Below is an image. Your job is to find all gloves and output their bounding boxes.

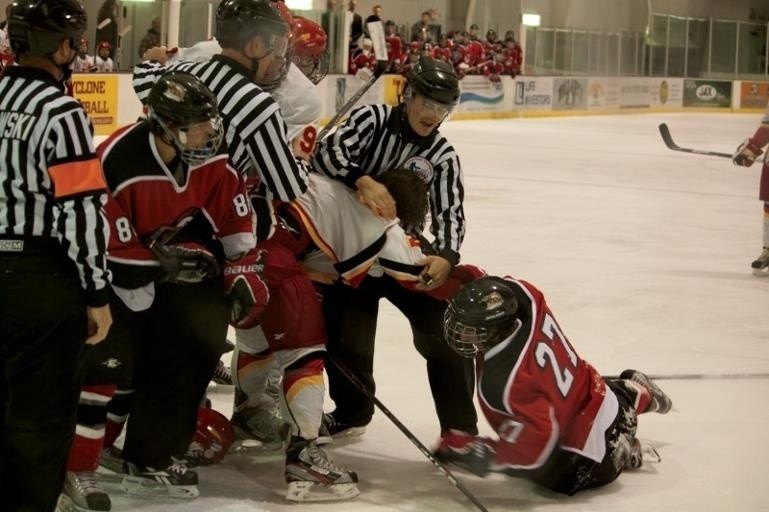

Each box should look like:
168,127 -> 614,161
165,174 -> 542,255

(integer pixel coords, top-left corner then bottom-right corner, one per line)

433,433 -> 494,478
174,242 -> 218,277
220,249 -> 270,329
732,137 -> 761,167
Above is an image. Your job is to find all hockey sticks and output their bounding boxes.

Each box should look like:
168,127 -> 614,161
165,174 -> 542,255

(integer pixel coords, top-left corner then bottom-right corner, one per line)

327,354 -> 489,512
314,15 -> 389,140
659,124 -> 764,164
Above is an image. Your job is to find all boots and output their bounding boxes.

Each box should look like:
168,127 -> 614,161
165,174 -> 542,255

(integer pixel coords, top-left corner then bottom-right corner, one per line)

123,458 -> 199,487
229,407 -> 290,444
751,247 -> 769,268
97,444 -> 127,472
324,408 -> 369,435
620,370 -> 671,414
62,469 -> 112,511
284,434 -> 359,484
212,361 -> 233,386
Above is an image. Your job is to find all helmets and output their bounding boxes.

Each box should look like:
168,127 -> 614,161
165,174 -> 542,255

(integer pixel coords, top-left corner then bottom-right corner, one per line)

98,42 -> 113,54
404,59 -> 460,104
80,39 -> 89,48
214,0 -> 294,46
290,15 -> 328,61
441,278 -> 519,358
6,0 -> 87,56
409,24 -> 515,54
148,71 -> 224,164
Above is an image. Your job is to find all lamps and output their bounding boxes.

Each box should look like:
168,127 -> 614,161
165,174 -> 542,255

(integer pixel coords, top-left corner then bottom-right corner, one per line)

521,11 -> 543,30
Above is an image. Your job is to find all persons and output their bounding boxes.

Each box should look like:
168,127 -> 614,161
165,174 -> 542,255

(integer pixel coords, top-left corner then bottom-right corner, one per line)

428,264 -> 672,493
734,103 -> 768,272
